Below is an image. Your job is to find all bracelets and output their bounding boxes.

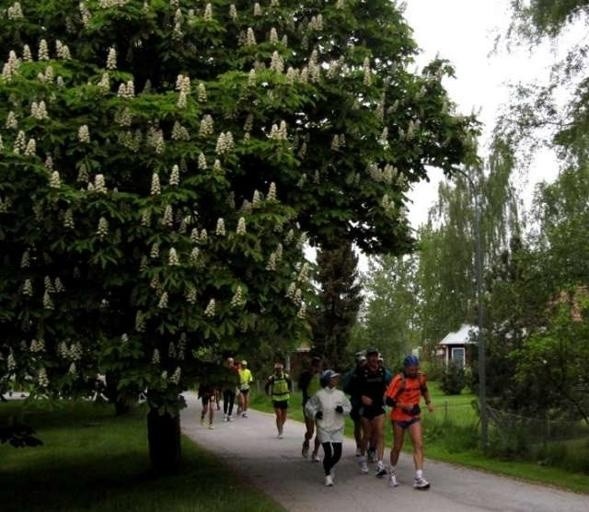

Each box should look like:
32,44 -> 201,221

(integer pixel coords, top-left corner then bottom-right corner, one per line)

425,400 -> 431,404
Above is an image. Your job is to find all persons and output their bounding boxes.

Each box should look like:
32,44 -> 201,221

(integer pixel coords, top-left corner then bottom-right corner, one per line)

196,356 -> 254,430
297,355 -> 323,464
383,354 -> 435,490
303,366 -> 353,487
342,346 -> 393,479
264,362 -> 296,440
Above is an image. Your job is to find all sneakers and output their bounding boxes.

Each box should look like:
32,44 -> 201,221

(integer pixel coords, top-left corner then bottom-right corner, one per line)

200,411 -> 249,430
302,441 -> 309,457
324,464 -> 336,486
312,450 -> 320,462
412,476 -> 430,490
277,432 -> 283,438
387,465 -> 400,487
356,446 -> 389,479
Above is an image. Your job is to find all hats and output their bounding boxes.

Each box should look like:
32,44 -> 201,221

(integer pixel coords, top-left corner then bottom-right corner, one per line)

321,369 -> 339,380
366,347 -> 379,356
354,352 -> 367,361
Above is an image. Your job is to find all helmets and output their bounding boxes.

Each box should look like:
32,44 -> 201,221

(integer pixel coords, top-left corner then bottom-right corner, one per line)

403,354 -> 419,366
